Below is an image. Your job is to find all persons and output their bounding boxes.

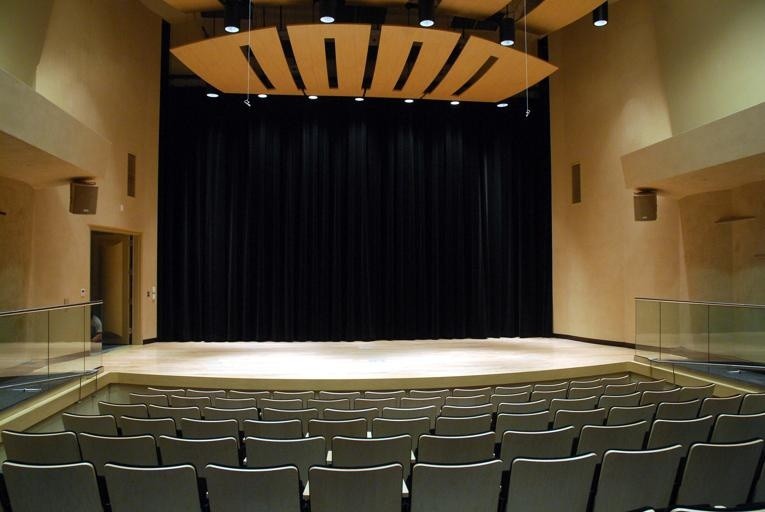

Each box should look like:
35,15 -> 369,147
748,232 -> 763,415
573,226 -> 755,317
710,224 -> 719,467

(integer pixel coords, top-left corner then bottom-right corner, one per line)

90,312 -> 102,342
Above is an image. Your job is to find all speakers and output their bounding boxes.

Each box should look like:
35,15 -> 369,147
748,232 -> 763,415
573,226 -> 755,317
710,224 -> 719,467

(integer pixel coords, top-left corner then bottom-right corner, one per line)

70,183 -> 99,215
633,192 -> 657,221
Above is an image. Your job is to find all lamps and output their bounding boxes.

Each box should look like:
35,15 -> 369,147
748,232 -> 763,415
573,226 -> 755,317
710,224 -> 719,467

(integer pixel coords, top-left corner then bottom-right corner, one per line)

319,0 -> 337,24
419,3 -> 437,31
592,2 -> 611,29
498,4 -> 516,48
224,2 -> 242,34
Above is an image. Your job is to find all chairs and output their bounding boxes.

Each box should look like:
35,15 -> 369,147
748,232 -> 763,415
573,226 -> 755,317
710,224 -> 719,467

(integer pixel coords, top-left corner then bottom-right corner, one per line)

272,391 -> 316,409
434,413 -> 493,437
370,405 -> 443,429
169,395 -> 212,417
606,382 -> 639,396
496,402 -> 549,416
308,398 -> 351,419
303,466 -> 410,512
204,406 -> 258,431
260,409 -> 319,439
569,379 -> 603,389
575,419 -> 651,457
712,412 -> 765,444
401,396 -> 445,419
159,435 -> 241,479
533,384 -> 570,393
506,452 -> 598,512
551,409 -> 606,430
494,411 -> 549,443
601,375 -> 631,386
740,394 -> 765,414
363,391 -> 406,409
530,389 -> 567,403
675,437 -> 765,512
638,380 -> 670,397
443,405 -> 491,416
332,435 -> 413,483
118,415 -> 178,447
647,415 -> 715,451
324,408 -> 379,434
128,392 -> 170,407
230,391 -> 270,409
98,399 -> 148,429
317,391 -> 362,410
640,388 -> 685,407
309,418 -> 369,450
215,397 -> 256,411
494,386 -> 532,397
678,385 -> 715,405
373,415 -> 433,450
242,419 -> 303,442
186,389 -> 226,407
262,398 -> 302,412
499,427 -> 577,472
353,399 -> 400,419
205,465 -> 300,512
146,404 -> 201,430
568,385 -> 605,406
453,389 -> 492,404
445,394 -> 487,408
607,404 -> 658,428
78,432 -> 159,476
600,392 -> 648,416
411,458 -> 506,512
549,396 -> 600,422
61,413 -> 120,437
0,461 -> 105,512
490,391 -> 529,412
656,399 -> 703,423
595,443 -> 683,512
0,428 -> 80,467
247,436 -> 329,488
148,387 -> 186,407
179,415 -> 240,451
699,394 -> 744,417
418,431 -> 496,467
408,389 -> 452,407
104,464 -> 202,512
